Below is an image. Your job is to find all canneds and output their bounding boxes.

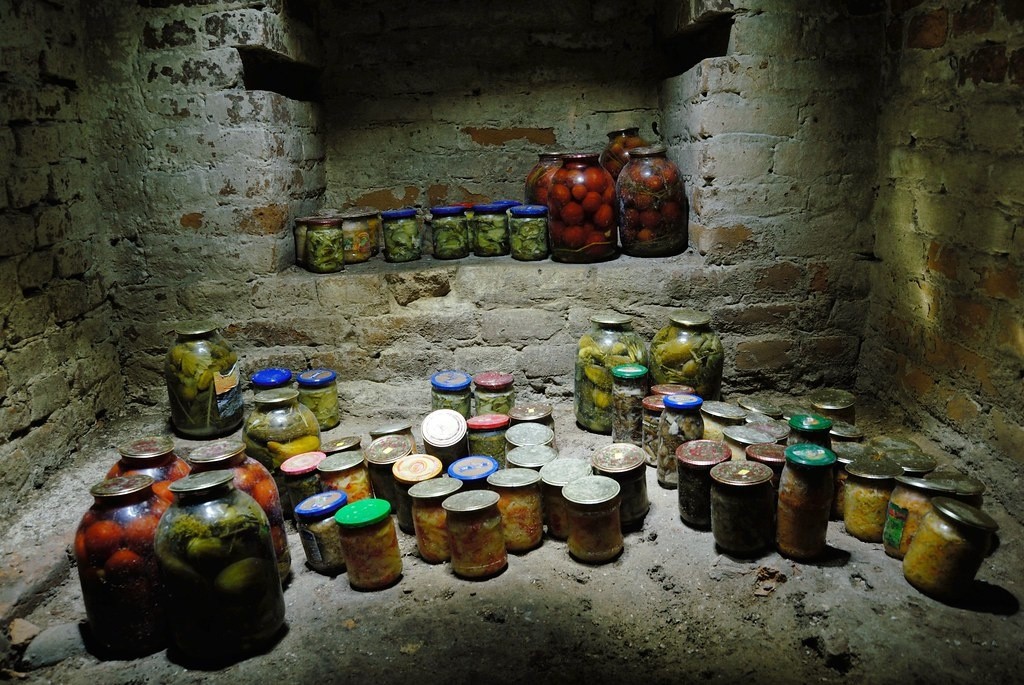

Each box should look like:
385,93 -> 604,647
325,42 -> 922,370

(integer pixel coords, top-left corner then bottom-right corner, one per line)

77,320 -> 995,662
295,127 -> 688,272
